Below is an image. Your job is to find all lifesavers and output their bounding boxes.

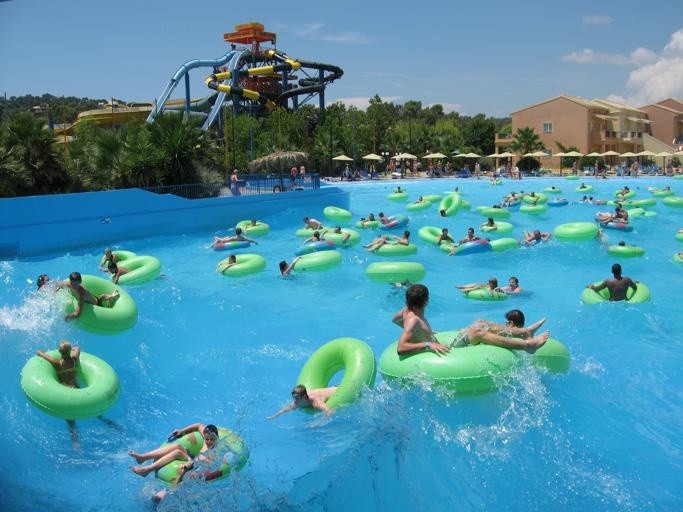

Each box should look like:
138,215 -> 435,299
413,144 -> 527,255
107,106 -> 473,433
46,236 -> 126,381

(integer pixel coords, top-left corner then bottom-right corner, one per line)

53,250 -> 161,335
217,253 -> 264,278
212,219 -> 269,251
292,226 -> 361,273
673,175 -> 683,179
406,184 -> 683,266
366,261 -> 425,285
567,176 -> 579,180
356,212 -> 417,256
388,188 -> 408,202
581,280 -> 651,307
460,285 -> 508,302
153,427 -> 250,486
20,348 -> 120,419
488,179 -> 502,185
295,331 -> 570,416
324,206 -> 352,223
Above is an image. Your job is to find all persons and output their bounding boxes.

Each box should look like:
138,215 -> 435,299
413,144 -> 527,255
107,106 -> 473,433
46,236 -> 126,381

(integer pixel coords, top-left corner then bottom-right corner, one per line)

493,276 -> 523,294
290,164 -> 298,186
368,212 -> 375,221
98,248 -> 121,272
455,277 -> 498,292
391,283 -> 550,364
250,218 -> 258,226
303,229 -> 322,244
585,263 -> 637,300
378,212 -> 389,224
209,225 -> 258,249
579,182 -> 633,246
478,216 -> 497,233
127,422 -> 219,479
571,159 -> 640,179
214,254 -> 236,272
475,309 -> 525,329
437,228 -> 455,244
522,227 -> 552,244
36,274 -> 51,289
54,271 -> 120,320
229,169 -> 241,196
475,161 -> 480,178
149,465 -> 185,503
394,183 -> 534,217
303,217 -> 322,230
34,341 -> 125,451
488,160 -> 522,186
108,262 -> 129,284
362,229 -> 411,251
462,227 -> 477,245
412,160 -> 453,180
266,384 -> 338,430
321,226 -> 351,244
279,255 -> 302,276
359,217 -> 368,227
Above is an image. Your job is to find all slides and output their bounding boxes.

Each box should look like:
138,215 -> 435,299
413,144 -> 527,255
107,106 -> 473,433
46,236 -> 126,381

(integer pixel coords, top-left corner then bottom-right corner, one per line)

205,50 -> 299,111
145,48 -> 250,141
278,57 -> 343,110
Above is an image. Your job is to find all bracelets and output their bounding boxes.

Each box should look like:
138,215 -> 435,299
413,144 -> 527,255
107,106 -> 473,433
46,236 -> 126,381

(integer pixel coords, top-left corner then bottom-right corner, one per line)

588,284 -> 593,289
424,341 -> 430,350
